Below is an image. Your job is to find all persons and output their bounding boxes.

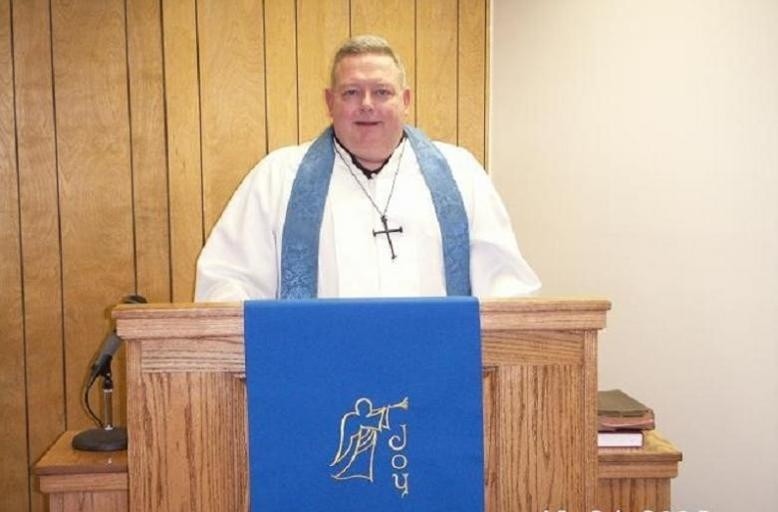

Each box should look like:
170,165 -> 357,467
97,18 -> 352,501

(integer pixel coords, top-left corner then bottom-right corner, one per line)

194,35 -> 542,302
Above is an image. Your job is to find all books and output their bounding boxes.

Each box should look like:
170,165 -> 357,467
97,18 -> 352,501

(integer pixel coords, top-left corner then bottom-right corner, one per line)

597,388 -> 655,448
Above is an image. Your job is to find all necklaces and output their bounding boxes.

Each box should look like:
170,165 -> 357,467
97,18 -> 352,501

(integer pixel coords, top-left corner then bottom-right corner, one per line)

332,134 -> 407,260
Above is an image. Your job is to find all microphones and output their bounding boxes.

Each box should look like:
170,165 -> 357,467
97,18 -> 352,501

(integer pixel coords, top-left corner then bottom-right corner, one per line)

86,294 -> 147,387
